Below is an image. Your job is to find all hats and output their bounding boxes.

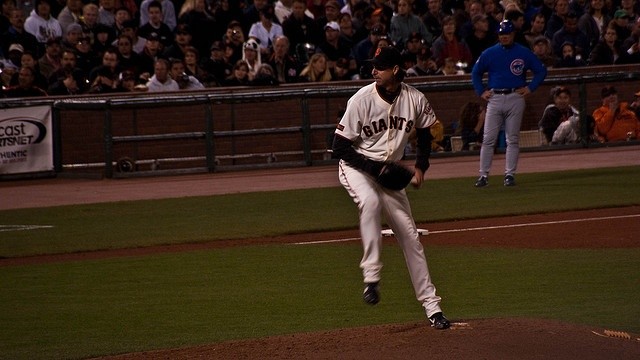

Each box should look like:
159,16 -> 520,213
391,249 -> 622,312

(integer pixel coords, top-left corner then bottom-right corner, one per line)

497,20 -> 514,32
600,85 -> 618,97
324,21 -> 340,30
408,30 -> 423,42
371,22 -> 386,36
243,37 -> 261,51
211,41 -> 226,53
364,47 -> 404,67
66,23 -> 83,35
470,14 -> 488,26
614,9 -> 629,18
325,1 -> 340,10
174,24 -> 191,34
8,43 -> 24,54
417,47 -> 434,61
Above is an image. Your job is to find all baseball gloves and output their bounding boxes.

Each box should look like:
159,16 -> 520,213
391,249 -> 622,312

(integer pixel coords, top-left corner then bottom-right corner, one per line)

358,155 -> 415,191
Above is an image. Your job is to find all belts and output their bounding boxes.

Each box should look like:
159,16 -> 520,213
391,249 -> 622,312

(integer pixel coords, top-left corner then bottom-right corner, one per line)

493,88 -> 516,95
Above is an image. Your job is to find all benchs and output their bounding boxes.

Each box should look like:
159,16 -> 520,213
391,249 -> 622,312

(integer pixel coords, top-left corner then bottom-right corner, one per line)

450,128 -> 545,153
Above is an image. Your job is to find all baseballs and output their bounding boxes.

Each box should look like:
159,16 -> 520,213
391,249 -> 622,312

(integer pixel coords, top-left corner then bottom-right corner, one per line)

411,176 -> 423,188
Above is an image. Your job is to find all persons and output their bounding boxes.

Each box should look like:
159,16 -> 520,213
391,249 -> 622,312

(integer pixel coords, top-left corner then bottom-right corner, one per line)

524,14 -> 551,46
313,0 -> 340,40
590,23 -> 629,65
551,11 -> 586,56
558,42 -> 587,67
406,47 -> 447,77
462,14 -> 493,73
100,46 -> 119,74
146,59 -> 179,91
206,41 -> 234,81
578,0 -> 609,42
503,4 -> 522,20
316,21 -> 342,60
608,9 -> 630,39
341,0 -> 372,14
24,1 -> 62,42
135,32 -> 169,77
339,13 -> 360,44
470,2 -> 484,21
592,87 -> 639,143
0,43 -> 26,71
267,36 -> 298,83
472,19 -> 549,189
298,53 -> 334,81
484,0 -> 504,21
112,22 -> 147,53
624,16 -> 640,49
618,0 -> 640,19
546,0 -> 570,37
400,32 -> 429,69
237,38 -> 263,81
222,20 -> 245,60
109,8 -> 138,42
629,88 -> 640,120
461,95 -> 488,144
408,115 -> 444,152
614,39 -> 639,64
162,24 -> 200,58
0,10 -> 42,64
432,15 -> 473,75
20,52 -> 37,75
59,23 -> 93,65
182,46 -> 208,88
504,8 -> 529,49
248,7 -> 284,54
331,57 -> 360,81
541,87 -> 582,144
48,49 -> 90,95
89,24 -> 118,67
531,36 -> 556,68
86,75 -> 113,93
76,4 -> 101,33
281,0 -> 317,54
179,0 -> 216,44
9,51 -> 49,93
350,24 -> 387,58
390,0 -> 434,46
242,0 -> 281,33
421,0 -> 447,36
48,69 -> 80,95
96,0 -> 118,25
251,63 -> 278,86
57,0 -> 83,33
138,1 -> 173,44
224,60 -> 251,86
140,0 -> 176,31
4,66 -> 49,97
0,0 -> 18,30
376,36 -> 389,52
117,35 -> 142,69
541,88 -> 580,126
274,0 -> 314,27
38,37 -> 65,77
170,59 -> 206,90
332,45 -> 451,330
119,69 -> 135,80
115,71 -> 148,92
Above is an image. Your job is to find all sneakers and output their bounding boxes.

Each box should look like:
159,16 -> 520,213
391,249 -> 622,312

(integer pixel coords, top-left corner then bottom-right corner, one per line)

475,175 -> 488,187
503,174 -> 514,186
429,313 -> 450,329
363,283 -> 381,305
